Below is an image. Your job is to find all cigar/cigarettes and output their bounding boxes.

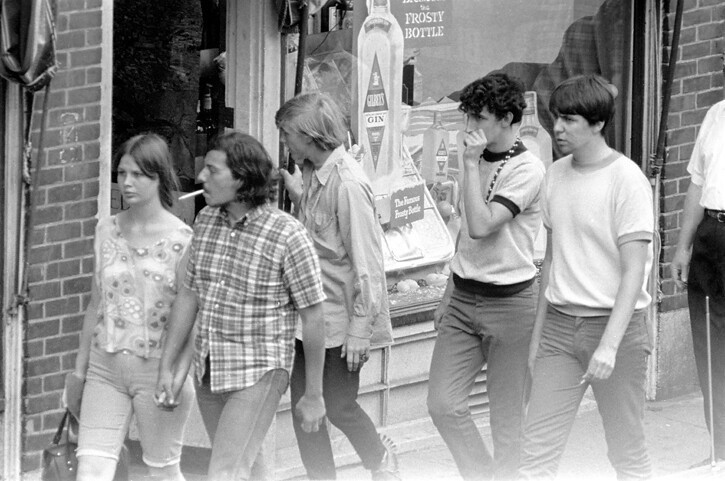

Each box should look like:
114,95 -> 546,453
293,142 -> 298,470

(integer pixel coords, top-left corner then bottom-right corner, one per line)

178,189 -> 204,200
581,379 -> 585,384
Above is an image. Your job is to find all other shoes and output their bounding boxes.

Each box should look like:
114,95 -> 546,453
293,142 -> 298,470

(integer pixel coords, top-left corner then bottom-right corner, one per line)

369,445 -> 396,480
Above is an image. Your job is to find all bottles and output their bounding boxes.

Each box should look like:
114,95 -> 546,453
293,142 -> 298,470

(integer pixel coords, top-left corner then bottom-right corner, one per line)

195,83 -> 219,156
421,110 -> 448,183
357,0 -> 404,194
517,90 -> 553,172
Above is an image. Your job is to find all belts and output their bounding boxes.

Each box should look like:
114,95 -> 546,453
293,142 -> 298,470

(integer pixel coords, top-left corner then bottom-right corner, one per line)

707,210 -> 725,223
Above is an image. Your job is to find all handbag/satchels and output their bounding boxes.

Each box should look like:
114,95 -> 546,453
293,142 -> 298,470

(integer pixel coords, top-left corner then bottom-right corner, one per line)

42,410 -> 131,481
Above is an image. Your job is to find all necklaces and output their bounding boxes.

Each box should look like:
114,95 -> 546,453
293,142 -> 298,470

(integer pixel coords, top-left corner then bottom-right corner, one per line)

478,136 -> 521,205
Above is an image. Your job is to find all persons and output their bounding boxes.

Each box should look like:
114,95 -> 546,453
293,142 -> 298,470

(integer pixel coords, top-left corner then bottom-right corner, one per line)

670,47 -> 725,469
61,132 -> 327,481
514,74 -> 654,481
274,90 -> 402,481
426,73 -> 547,481
